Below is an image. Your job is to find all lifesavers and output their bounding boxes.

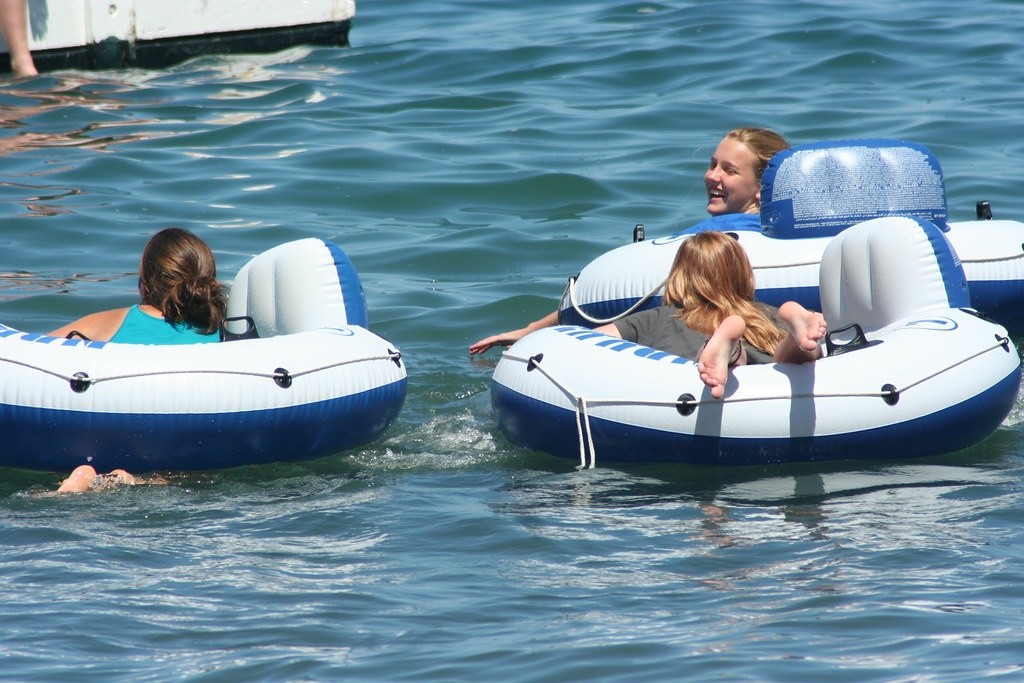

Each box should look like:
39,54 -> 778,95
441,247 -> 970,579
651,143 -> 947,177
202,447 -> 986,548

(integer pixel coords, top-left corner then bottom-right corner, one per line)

558,139 -> 1024,323
490,216 -> 1022,464
0,234 -> 409,475
4,3 -> 359,62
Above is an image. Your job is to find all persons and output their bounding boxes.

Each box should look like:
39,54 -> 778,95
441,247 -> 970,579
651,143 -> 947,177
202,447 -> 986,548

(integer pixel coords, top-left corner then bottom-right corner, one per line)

592,232 -> 827,399
0,0 -> 37,75
470,129 -> 789,354
47,227 -> 228,492
